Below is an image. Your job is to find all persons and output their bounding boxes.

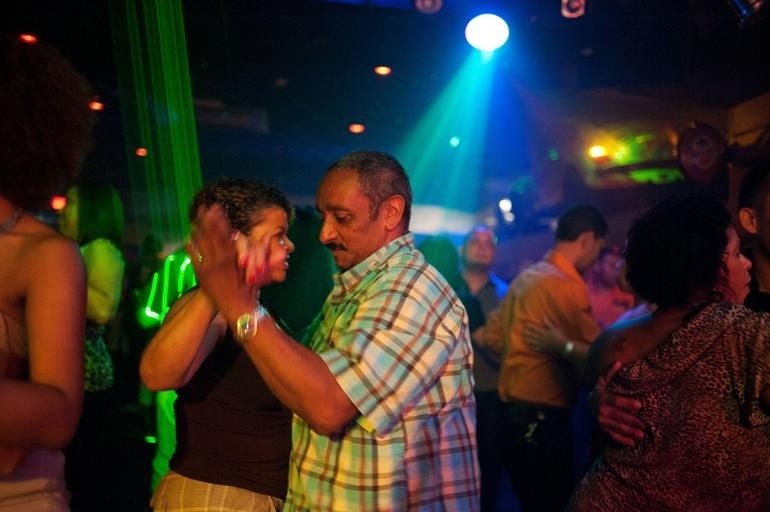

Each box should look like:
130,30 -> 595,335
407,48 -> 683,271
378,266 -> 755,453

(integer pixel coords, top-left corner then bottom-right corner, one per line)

1,38 -> 770,511
